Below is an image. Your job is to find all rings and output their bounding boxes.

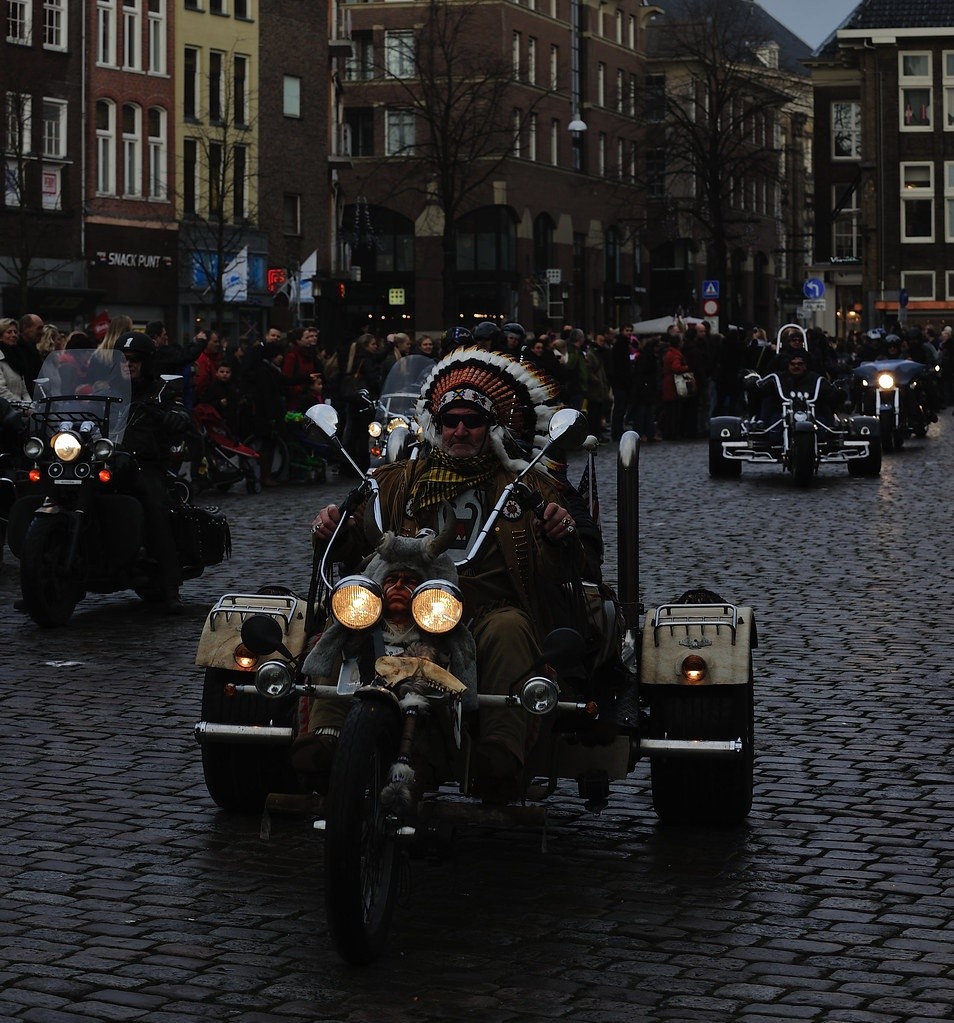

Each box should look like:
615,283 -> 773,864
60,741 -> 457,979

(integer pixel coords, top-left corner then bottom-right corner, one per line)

316,521 -> 323,529
311,527 -> 317,534
561,517 -> 569,527
567,525 -> 575,533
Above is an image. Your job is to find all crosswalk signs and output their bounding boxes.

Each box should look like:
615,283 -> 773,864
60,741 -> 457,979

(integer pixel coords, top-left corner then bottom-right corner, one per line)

699,279 -> 722,300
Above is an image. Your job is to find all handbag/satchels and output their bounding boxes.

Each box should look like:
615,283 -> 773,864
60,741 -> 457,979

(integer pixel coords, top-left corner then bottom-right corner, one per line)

674,371 -> 696,397
343,359 -> 380,397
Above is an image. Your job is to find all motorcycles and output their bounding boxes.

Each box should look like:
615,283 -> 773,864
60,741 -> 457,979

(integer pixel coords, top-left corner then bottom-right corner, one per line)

1,348 -> 235,631
846,359 -> 941,446
708,370 -> 883,488
354,354 -> 439,478
187,400 -> 761,966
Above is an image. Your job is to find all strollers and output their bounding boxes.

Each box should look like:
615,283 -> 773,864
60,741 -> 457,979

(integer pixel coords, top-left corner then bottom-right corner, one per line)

190,400 -> 265,498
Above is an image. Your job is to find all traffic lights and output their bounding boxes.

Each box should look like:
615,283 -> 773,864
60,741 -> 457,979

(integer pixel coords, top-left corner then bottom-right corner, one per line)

331,278 -> 350,303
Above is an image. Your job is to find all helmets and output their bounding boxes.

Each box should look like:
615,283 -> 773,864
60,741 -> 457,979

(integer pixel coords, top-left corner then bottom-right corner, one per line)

113,331 -> 158,362
475,321 -> 500,338
866,329 -> 881,339
877,328 -> 887,334
885,334 -> 902,349
452,327 -> 476,345
780,327 -> 804,344
502,322 -> 526,338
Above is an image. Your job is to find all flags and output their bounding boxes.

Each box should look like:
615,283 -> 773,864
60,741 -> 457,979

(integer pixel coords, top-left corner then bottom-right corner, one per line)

577,452 -> 602,530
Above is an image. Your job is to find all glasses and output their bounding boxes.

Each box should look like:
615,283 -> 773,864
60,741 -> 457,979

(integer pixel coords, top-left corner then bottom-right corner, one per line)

625,328 -> 632,332
791,362 -> 803,366
440,412 -> 489,432
120,354 -> 140,364
792,337 -> 803,342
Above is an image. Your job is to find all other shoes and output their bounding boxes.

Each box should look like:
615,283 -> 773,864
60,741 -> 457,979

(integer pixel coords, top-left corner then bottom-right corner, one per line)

587,431 -> 610,446
647,436 -> 663,444
639,437 -> 648,445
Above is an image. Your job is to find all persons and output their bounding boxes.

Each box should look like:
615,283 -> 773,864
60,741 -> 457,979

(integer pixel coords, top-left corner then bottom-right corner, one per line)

305,493 -> 479,709
0,309 -> 953,576
291,346 -> 604,797
